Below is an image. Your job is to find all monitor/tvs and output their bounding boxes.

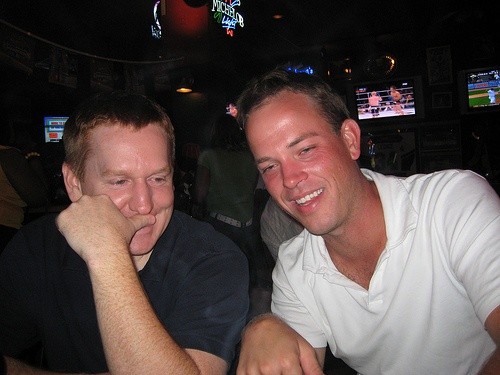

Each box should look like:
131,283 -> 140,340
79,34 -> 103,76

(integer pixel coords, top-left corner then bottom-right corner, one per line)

465,67 -> 500,108
353,78 -> 416,120
44,117 -> 70,143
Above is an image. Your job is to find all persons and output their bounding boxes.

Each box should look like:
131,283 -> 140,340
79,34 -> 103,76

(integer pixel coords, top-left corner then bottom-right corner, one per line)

488,88 -> 496,104
0,91 -> 250,375
390,86 -> 404,114
235,67 -> 500,375
493,73 -> 500,86
369,92 -> 382,117
193,114 -> 258,271
